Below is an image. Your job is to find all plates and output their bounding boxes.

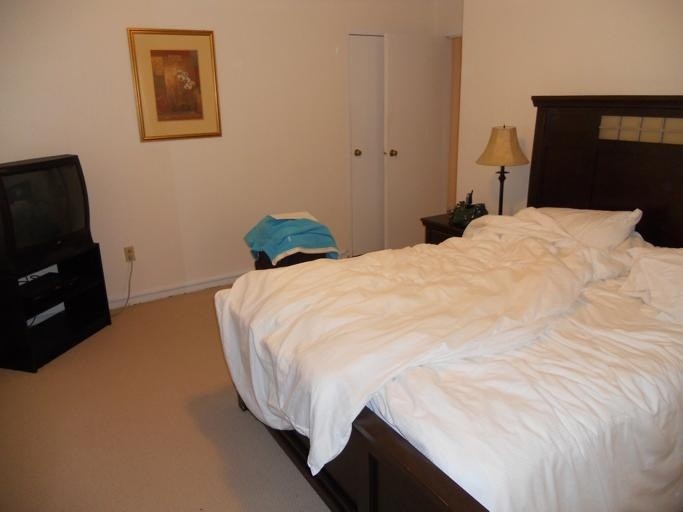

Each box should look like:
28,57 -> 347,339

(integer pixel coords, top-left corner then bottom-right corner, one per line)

0,242 -> 113,373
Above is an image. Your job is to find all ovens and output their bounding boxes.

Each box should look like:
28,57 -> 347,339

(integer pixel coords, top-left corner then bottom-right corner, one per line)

212,91 -> 681,511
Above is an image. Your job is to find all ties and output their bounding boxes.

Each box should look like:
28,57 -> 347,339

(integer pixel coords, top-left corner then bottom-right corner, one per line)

0,153 -> 94,271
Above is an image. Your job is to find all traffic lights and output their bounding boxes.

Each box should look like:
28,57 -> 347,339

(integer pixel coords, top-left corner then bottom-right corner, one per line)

619,246 -> 681,319
535,207 -> 643,250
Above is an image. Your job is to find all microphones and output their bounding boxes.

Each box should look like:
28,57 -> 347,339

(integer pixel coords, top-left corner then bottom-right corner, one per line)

252,245 -> 325,270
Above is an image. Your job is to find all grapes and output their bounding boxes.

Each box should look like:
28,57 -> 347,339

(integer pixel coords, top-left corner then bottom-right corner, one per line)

420,211 -> 485,246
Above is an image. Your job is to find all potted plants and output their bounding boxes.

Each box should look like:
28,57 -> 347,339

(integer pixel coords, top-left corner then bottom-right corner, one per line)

125,27 -> 223,144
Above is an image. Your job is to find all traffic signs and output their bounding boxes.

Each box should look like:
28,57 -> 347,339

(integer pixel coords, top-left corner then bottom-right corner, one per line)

122,247 -> 136,263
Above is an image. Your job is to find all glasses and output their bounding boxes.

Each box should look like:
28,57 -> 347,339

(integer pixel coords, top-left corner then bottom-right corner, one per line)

475,124 -> 531,215
